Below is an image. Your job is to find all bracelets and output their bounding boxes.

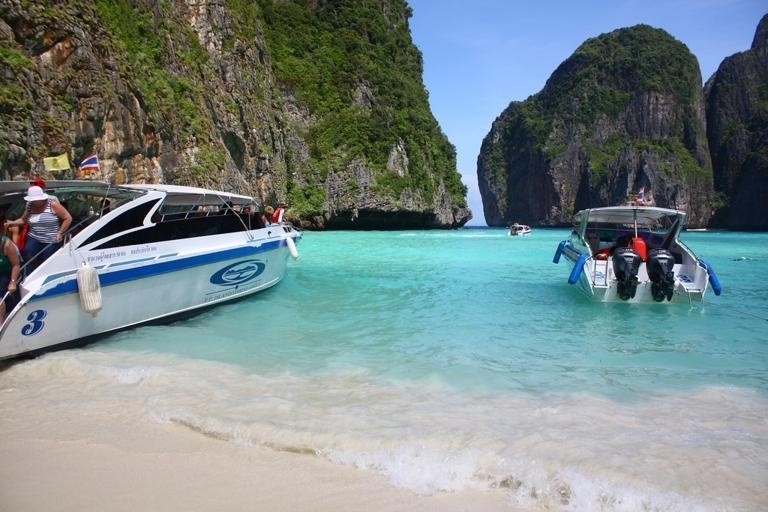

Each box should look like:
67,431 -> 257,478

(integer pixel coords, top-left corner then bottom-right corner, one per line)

10,279 -> 17,283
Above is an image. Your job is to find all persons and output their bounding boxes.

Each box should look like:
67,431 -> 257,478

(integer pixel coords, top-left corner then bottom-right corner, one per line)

270,204 -> 288,225
244,206 -> 253,216
6,178 -> 48,251
262,206 -> 274,226
2,186 -> 72,277
1,235 -> 26,322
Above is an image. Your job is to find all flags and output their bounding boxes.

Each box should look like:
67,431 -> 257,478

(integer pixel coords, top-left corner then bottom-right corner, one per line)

43,152 -> 71,173
78,151 -> 99,172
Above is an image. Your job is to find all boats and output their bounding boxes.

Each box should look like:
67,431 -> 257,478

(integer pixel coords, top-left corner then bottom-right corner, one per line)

0,170 -> 304,362
507,223 -> 531,238
556,203 -> 724,314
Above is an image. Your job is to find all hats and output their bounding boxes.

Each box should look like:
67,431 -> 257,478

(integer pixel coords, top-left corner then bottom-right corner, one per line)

264,206 -> 274,213
24,178 -> 49,201
277,203 -> 288,208
